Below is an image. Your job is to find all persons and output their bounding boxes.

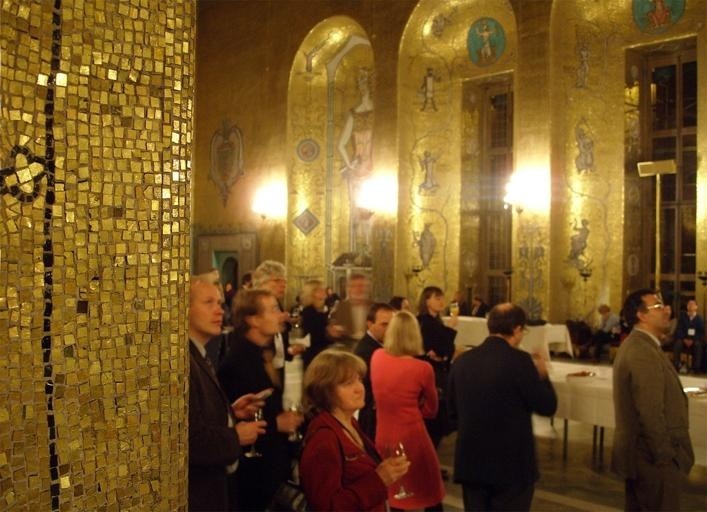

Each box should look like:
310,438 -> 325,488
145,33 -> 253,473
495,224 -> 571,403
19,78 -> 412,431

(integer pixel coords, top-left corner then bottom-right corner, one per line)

414,285 -> 458,448
298,348 -> 411,512
583,305 -> 630,363
224,282 -> 235,303
188,277 -> 267,512
327,275 -> 375,341
389,296 -> 412,312
369,312 -> 446,512
240,273 -> 252,290
301,280 -> 331,367
442,302 -> 559,512
252,260 -> 304,394
472,297 -> 490,318
216,289 -> 304,489
353,303 -> 395,438
325,286 -> 341,309
609,289 -> 695,512
661,299 -> 703,374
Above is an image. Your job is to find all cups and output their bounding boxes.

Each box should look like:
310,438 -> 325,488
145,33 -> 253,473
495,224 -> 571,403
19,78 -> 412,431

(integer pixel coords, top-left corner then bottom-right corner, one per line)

384,438 -> 415,499
449,302 -> 459,320
244,408 -> 263,458
287,400 -> 304,440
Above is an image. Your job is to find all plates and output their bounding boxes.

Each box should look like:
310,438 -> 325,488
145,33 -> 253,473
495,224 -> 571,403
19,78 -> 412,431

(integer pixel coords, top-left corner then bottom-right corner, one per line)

566,372 -> 596,382
683,387 -> 707,397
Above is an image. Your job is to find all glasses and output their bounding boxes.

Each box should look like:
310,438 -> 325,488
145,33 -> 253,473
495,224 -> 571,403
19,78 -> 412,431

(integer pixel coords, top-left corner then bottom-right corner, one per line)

270,278 -> 288,283
522,325 -> 531,334
646,302 -> 666,311
